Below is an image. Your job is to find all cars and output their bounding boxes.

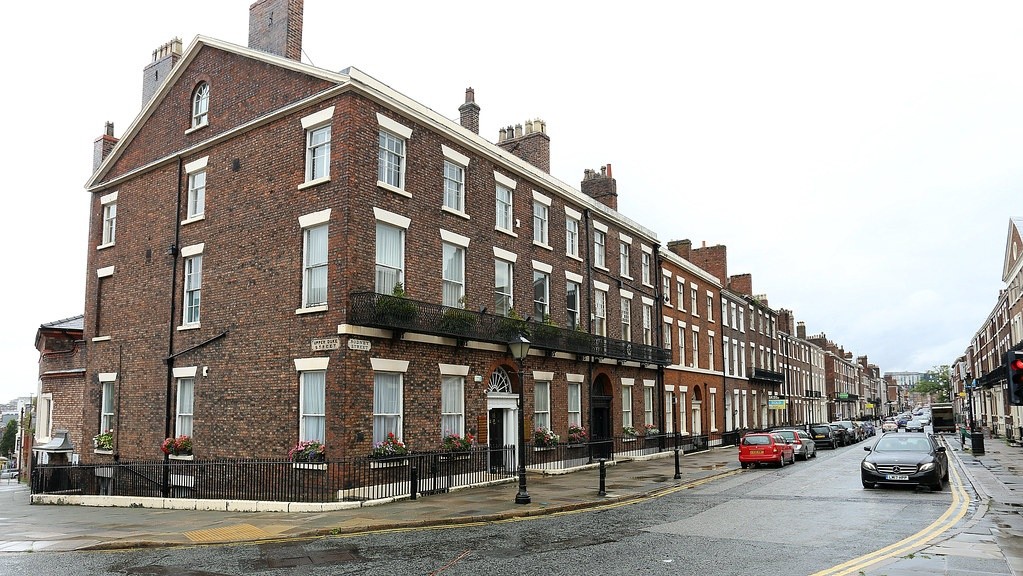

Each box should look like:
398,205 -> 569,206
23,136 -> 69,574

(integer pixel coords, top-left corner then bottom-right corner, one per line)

882,421 -> 899,432
912,404 -> 932,425
897,414 -> 912,428
905,421 -> 924,433
829,424 -> 851,446
836,421 -> 876,443
769,429 -> 817,461
11,461 -> 17,468
861,432 -> 949,491
809,426 -> 838,450
2,469 -> 19,479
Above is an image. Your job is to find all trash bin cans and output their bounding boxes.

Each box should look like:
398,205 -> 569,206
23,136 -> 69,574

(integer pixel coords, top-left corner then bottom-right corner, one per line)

972,431 -> 985,453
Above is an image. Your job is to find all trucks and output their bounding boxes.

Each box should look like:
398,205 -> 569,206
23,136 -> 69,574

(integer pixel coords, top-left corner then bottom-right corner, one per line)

930,403 -> 956,433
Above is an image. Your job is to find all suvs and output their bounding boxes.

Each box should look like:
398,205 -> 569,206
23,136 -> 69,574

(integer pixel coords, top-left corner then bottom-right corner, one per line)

739,431 -> 794,468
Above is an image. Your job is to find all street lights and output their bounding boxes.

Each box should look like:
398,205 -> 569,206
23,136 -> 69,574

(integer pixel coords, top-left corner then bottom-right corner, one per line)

507,331 -> 532,504
964,373 -> 974,433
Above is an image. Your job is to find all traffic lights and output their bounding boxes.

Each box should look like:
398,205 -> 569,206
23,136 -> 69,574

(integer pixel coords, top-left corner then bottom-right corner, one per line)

1006,350 -> 1023,406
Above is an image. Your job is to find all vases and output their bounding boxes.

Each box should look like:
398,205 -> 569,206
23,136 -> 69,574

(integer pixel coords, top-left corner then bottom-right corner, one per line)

375,452 -> 408,463
295,450 -> 325,462
171,448 -> 192,456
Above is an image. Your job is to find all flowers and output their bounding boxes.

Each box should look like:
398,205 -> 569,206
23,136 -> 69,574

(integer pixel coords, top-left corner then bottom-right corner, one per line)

644,424 -> 660,434
368,432 -> 410,459
569,423 -> 588,444
160,433 -> 192,455
535,425 -> 559,447
440,427 -> 476,451
93,429 -> 114,451
288,439 -> 325,462
